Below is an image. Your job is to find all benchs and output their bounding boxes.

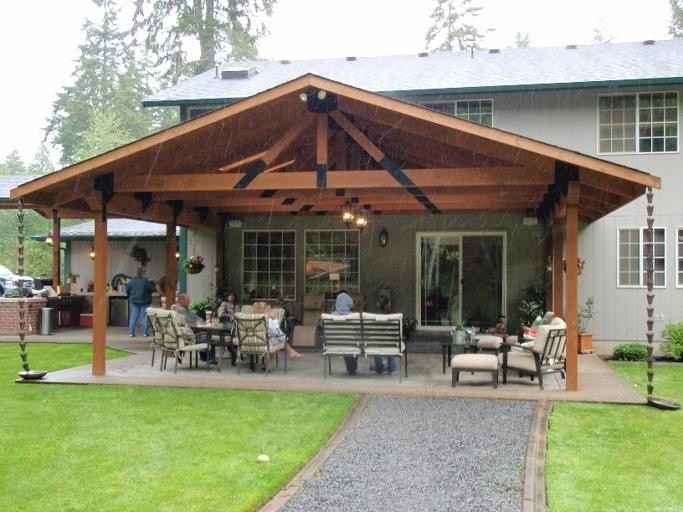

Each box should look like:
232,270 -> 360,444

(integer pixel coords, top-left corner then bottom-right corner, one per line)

320,313 -> 407,383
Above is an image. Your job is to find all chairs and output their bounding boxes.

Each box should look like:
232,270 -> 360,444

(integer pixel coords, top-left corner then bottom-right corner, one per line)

154,313 -> 212,374
496,329 -> 566,389
521,317 -> 566,350
145,310 -> 189,366
233,314 -> 288,378
518,312 -> 554,342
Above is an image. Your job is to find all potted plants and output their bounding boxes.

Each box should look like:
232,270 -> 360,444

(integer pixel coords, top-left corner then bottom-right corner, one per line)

563,257 -> 584,276
181,255 -> 205,274
578,296 -> 595,354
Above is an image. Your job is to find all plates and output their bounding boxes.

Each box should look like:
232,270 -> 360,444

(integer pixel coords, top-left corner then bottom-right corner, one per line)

648,397 -> 680,409
18,370 -> 48,379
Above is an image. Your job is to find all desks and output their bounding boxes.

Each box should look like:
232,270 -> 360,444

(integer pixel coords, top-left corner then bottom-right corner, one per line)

440,338 -> 508,373
186,321 -> 265,373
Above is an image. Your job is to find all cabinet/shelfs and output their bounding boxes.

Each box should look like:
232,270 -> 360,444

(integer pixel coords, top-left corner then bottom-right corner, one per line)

303,295 -> 364,326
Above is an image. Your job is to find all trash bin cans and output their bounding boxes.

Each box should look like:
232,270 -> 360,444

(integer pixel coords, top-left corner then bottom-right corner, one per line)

39,308 -> 54,336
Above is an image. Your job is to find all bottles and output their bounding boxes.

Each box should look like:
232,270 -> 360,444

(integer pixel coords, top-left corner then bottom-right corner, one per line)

107,282 -> 109,289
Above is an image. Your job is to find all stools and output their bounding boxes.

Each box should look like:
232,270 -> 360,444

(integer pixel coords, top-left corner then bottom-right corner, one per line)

451,354 -> 499,388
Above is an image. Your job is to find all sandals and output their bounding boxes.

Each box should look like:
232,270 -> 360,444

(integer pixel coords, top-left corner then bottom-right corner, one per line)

289,352 -> 303,359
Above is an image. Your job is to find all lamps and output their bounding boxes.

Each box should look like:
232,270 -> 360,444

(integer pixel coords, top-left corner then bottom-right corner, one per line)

90,237 -> 96,259
355,209 -> 369,233
380,226 -> 388,246
342,203 -> 355,227
45,232 -> 53,245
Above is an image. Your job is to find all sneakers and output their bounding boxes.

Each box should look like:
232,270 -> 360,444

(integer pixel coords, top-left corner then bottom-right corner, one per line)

209,361 -> 218,365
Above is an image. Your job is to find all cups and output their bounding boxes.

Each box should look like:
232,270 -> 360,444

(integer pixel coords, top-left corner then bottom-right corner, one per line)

206,310 -> 212,323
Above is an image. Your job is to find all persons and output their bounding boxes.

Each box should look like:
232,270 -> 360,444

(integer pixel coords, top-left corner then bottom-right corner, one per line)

219,290 -> 238,323
370,295 -> 398,374
171,294 -> 217,366
253,302 -> 304,372
126,267 -> 157,337
331,292 -> 361,375
